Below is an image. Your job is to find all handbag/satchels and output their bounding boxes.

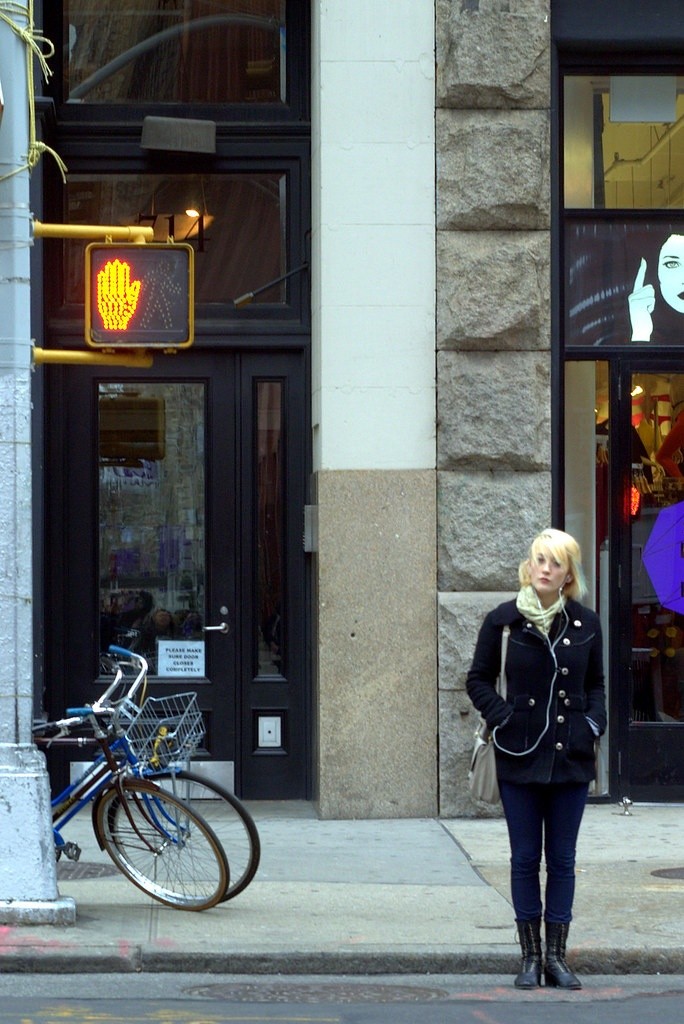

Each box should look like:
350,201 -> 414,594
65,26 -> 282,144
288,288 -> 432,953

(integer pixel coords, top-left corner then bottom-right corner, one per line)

467,715 -> 501,805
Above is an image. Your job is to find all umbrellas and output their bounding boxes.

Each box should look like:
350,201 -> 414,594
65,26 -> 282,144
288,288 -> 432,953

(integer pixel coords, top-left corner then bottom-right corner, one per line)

642,502 -> 684,615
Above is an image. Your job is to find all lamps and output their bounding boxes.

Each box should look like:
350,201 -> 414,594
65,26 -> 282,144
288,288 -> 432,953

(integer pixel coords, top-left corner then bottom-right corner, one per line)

184,190 -> 206,220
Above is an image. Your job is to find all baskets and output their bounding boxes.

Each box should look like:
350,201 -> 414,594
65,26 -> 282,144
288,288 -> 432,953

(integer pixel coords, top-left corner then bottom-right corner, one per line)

124,691 -> 206,773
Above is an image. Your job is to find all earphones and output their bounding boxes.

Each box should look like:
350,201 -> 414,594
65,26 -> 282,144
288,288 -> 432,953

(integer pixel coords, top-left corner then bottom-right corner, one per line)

562,575 -> 570,585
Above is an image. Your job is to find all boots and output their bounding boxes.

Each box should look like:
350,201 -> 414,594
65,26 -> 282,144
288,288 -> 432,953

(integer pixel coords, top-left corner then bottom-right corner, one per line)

515,918 -> 542,988
543,920 -> 581,989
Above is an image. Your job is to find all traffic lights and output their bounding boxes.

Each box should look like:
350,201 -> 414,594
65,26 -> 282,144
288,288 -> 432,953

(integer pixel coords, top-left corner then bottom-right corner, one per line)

84,242 -> 195,348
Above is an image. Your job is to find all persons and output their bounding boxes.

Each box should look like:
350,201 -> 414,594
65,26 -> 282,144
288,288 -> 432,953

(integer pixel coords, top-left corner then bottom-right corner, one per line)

133,609 -> 202,673
627,233 -> 684,346
464,527 -> 607,990
630,421 -> 666,485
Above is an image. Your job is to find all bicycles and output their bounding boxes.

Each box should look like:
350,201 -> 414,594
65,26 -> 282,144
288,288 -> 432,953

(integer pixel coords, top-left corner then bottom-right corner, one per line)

31,645 -> 261,911
